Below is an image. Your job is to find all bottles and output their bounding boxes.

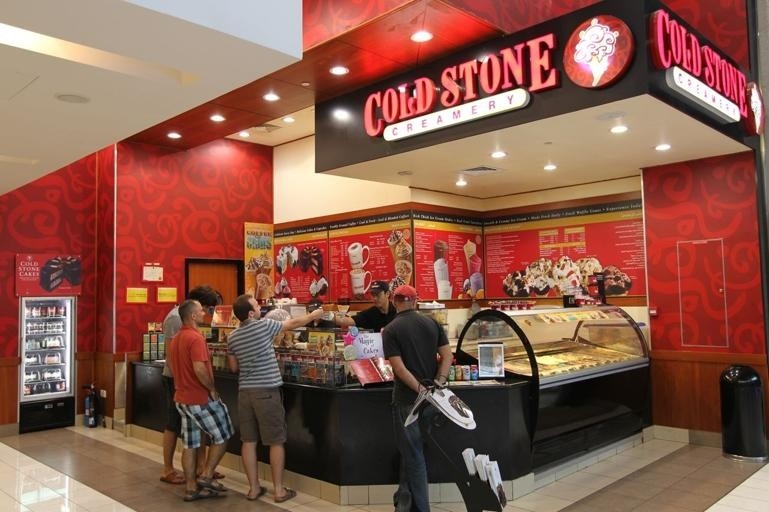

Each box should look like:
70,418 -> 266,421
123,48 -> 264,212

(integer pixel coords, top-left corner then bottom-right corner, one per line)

208,343 -> 230,373
273,351 -> 342,388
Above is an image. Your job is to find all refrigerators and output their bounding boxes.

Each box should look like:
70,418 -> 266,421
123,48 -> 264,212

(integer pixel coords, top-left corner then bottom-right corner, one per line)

13,293 -> 76,435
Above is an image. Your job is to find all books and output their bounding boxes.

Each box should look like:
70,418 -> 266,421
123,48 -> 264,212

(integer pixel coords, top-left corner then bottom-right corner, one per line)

348,357 -> 394,387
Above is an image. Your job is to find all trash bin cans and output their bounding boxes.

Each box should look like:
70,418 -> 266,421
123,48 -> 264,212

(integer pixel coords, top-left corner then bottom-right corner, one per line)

719,364 -> 768,464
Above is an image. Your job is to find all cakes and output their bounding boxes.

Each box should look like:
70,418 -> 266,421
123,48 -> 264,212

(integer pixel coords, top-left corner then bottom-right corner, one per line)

40,255 -> 81,291
502,255 -> 631,298
274,245 -> 328,298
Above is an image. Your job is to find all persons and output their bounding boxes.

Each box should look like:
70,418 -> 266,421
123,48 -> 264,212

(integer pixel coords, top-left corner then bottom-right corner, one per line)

297,299 -> 341,342
167,299 -> 219,502
382,285 -> 454,512
226,295 -> 324,503
322,280 -> 398,332
159,285 -> 225,484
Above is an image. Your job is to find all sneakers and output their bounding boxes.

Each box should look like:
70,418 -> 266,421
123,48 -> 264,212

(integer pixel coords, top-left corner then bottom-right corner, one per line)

391,488 -> 414,512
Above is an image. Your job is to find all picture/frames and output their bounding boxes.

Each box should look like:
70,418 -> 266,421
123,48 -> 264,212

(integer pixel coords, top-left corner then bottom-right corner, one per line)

477,343 -> 505,378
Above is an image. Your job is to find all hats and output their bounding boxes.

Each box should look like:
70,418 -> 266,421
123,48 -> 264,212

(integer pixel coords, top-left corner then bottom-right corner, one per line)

366,279 -> 389,295
393,284 -> 418,299
307,298 -> 321,311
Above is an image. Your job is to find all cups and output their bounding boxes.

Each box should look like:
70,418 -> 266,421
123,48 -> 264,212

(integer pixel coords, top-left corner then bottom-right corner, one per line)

347,242 -> 370,270
388,232 -> 411,285
22,303 -> 65,318
490,302 -> 535,312
576,298 -> 601,305
245,256 -> 272,300
431,239 -> 485,299
337,304 -> 350,313
349,268 -> 372,295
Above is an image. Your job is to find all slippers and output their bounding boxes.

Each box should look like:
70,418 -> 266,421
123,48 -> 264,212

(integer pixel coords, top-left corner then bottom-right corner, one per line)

275,483 -> 298,503
159,469 -> 186,484
195,467 -> 226,479
246,482 -> 268,501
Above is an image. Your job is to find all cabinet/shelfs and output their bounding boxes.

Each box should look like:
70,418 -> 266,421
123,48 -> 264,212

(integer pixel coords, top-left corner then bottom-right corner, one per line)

455,303 -> 653,473
205,326 -> 375,388
417,306 -> 450,338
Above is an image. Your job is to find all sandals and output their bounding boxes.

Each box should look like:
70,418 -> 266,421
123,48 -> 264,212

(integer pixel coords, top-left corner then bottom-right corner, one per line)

183,488 -> 218,501
196,474 -> 228,492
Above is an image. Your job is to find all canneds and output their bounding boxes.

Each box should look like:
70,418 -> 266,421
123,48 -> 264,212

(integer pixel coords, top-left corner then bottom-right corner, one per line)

563,272 -> 606,308
448,364 -> 478,381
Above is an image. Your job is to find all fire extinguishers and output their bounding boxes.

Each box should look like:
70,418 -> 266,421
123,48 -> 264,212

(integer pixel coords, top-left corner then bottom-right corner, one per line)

82,382 -> 106,429
